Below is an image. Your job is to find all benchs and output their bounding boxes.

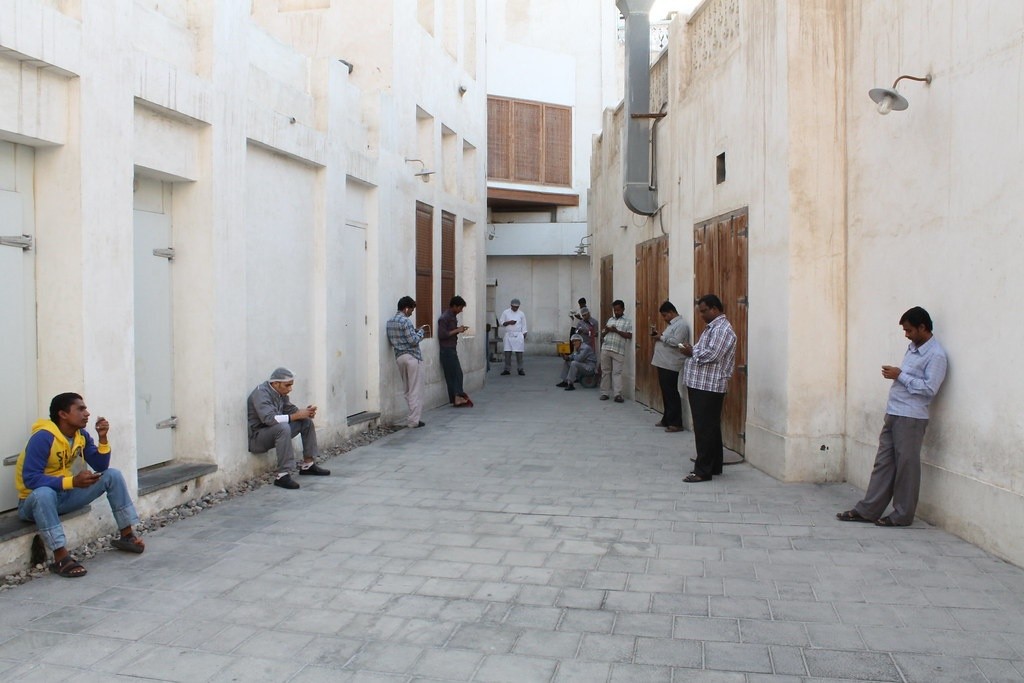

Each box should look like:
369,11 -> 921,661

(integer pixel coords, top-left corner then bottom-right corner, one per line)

347,411 -> 381,427
138,461 -> 217,498
0,505 -> 92,545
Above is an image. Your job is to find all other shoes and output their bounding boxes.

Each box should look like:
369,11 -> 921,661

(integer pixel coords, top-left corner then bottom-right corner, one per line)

665,426 -> 683,432
555,380 -> 568,387
500,370 -> 511,375
274,473 -> 300,489
682,471 -> 707,482
565,382 -> 575,390
416,421 -> 425,428
614,395 -> 624,402
518,368 -> 525,375
655,421 -> 664,427
600,395 -> 609,400
453,391 -> 474,407
298,462 -> 330,476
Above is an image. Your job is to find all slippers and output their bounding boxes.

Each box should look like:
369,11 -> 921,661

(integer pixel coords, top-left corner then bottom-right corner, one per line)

110,533 -> 144,553
874,515 -> 905,526
836,509 -> 874,523
49,556 -> 86,577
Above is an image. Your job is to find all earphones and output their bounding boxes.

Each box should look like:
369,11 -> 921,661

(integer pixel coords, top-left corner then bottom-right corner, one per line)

405,308 -> 408,313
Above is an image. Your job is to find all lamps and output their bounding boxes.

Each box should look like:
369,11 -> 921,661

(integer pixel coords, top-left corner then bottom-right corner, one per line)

869,74 -> 932,115
405,156 -> 436,182
574,233 -> 593,257
486,218 -> 500,240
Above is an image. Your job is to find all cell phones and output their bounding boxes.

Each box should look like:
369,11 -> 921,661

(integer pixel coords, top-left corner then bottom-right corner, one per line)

678,343 -> 685,348
94,472 -> 103,475
652,332 -> 663,335
513,321 -> 516,324
418,325 -> 426,329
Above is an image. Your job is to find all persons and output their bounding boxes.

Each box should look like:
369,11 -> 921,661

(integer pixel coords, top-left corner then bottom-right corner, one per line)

568,298 -> 598,354
651,302 -> 689,431
600,300 -> 632,401
437,296 -> 472,407
499,298 -> 527,376
16,392 -> 144,576
247,368 -> 330,489
387,296 -> 425,428
680,295 -> 737,482
836,307 -> 946,526
556,334 -> 597,391
